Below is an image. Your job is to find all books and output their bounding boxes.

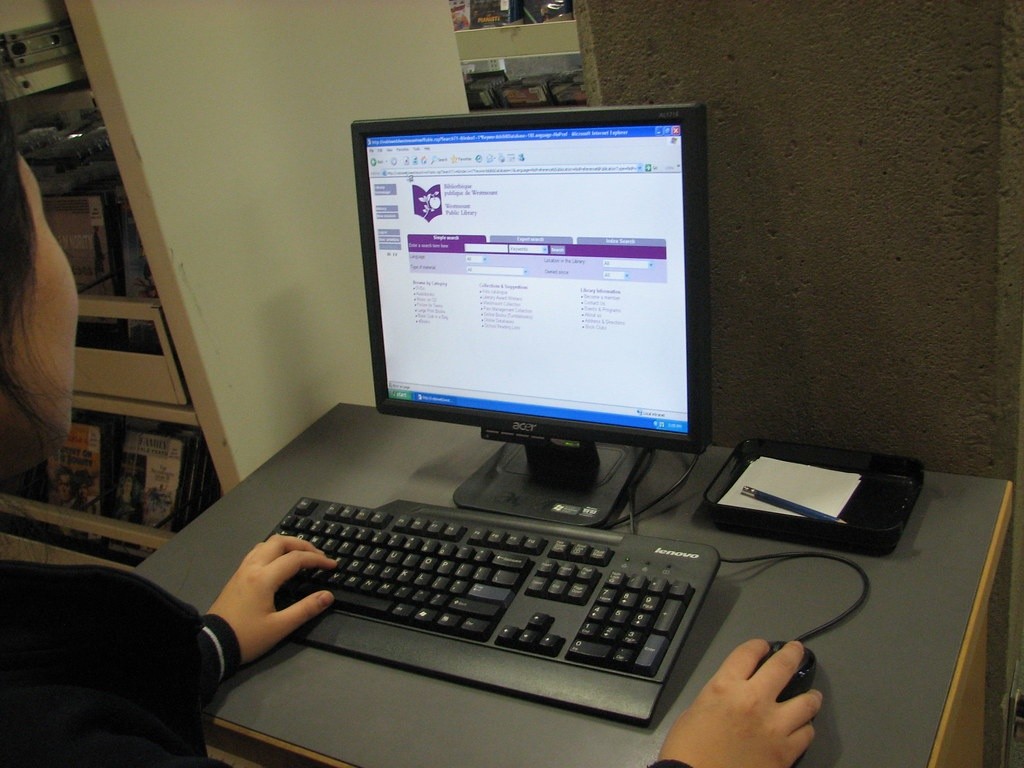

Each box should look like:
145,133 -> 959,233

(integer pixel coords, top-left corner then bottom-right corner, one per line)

467,70 -> 585,106
45,413 -> 222,534
449,0 -> 573,28
44,190 -> 160,298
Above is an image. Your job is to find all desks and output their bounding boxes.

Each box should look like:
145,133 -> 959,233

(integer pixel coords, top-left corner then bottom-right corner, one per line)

130,402 -> 1014,768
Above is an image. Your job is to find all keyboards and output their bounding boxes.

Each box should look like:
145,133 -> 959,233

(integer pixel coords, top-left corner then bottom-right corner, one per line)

261,497 -> 720,730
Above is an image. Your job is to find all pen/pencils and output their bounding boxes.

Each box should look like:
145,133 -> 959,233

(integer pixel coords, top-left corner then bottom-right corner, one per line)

742,486 -> 847,524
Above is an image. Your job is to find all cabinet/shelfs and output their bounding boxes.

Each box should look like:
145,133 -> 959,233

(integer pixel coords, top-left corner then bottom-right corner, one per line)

0,0 -> 583,567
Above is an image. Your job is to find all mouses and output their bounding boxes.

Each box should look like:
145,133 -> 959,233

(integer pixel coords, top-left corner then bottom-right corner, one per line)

749,639 -> 817,703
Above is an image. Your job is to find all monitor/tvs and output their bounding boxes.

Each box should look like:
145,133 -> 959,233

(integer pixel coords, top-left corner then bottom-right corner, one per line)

351,102 -> 714,526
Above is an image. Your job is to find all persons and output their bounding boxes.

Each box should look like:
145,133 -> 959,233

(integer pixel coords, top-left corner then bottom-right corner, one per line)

0,89 -> 823,768
